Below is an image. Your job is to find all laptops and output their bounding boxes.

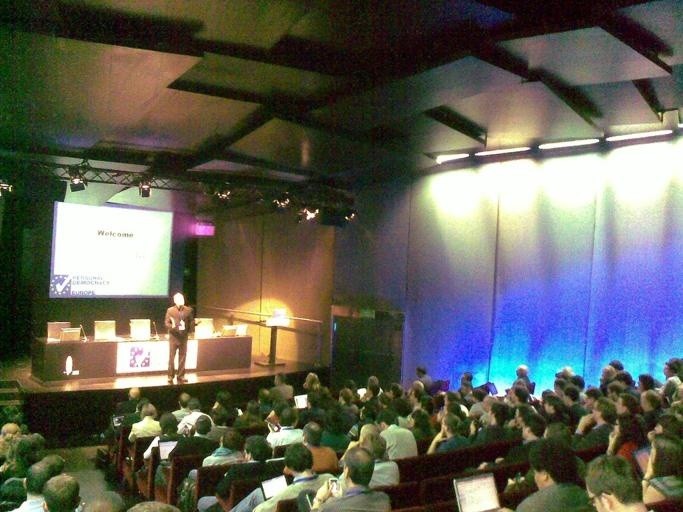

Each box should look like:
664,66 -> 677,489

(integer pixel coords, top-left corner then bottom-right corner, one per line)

488,382 -> 502,397
633,446 -> 651,473
294,393 -> 310,412
158,438 -> 178,460
452,472 -> 510,512
260,475 -> 288,501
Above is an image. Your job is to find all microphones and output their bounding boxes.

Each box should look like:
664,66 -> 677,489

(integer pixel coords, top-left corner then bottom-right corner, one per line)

79,324 -> 89,340
152,321 -> 160,340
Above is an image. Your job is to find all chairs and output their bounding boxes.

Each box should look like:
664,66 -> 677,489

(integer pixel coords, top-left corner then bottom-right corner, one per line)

194,317 -> 214,336
46,319 -> 152,339
114,425 -> 602,512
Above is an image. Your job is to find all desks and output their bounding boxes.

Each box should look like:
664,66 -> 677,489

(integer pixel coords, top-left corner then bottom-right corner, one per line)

29,334 -> 253,388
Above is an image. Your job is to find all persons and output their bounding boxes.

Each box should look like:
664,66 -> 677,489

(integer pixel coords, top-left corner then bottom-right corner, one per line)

1,406 -> 85,512
164,291 -> 195,385
98,356 -> 683,512
81,491 -> 128,512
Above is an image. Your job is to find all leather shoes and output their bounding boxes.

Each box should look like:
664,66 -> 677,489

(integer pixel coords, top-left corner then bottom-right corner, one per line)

168,376 -> 188,382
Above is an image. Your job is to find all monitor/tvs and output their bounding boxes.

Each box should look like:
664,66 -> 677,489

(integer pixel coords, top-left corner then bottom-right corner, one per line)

222,326 -> 238,337
60,328 -> 80,342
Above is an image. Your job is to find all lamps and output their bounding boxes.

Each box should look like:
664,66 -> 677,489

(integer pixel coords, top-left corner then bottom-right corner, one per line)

141,183 -> 151,198
70,177 -> 85,192
